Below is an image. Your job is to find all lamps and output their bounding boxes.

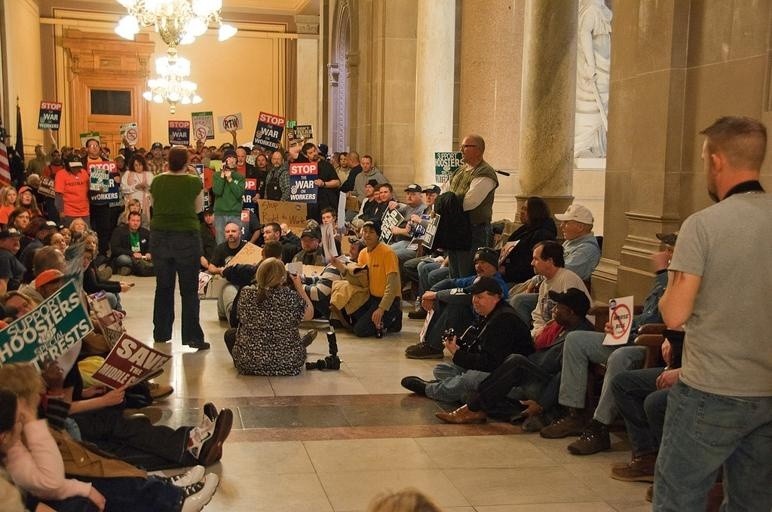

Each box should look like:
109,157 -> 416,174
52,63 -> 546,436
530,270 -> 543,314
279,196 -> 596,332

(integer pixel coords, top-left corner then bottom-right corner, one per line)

111,0 -> 241,65
142,57 -> 202,114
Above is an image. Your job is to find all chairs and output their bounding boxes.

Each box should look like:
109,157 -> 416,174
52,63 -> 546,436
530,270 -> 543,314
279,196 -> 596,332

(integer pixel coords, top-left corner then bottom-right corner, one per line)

587,304 -> 659,412
626,335 -> 664,462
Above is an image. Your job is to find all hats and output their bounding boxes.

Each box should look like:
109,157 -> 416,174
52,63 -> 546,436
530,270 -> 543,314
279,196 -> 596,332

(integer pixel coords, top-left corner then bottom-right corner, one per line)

0,227 -> 22,240
37,219 -> 60,231
66,153 -> 82,169
460,275 -> 503,297
553,202 -> 596,226
546,286 -> 592,316
32,268 -> 66,289
300,227 -> 322,241
151,142 -> 164,150
317,143 -> 330,155
404,183 -> 422,193
222,150 -> 238,163
654,226 -> 682,248
362,216 -> 383,237
420,184 -> 441,194
17,185 -> 35,194
222,143 -> 235,151
471,246 -> 501,272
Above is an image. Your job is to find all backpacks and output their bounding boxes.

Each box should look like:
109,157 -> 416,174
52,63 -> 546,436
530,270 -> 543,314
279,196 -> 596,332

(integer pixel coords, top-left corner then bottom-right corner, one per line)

264,168 -> 288,201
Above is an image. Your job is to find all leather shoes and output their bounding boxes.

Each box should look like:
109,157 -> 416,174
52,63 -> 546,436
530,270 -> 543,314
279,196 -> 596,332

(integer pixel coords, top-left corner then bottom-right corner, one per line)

432,402 -> 490,424
400,374 -> 441,397
519,411 -> 555,432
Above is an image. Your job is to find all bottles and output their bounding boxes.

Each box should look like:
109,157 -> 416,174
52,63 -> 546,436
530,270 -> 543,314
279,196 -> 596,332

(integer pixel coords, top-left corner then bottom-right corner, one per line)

374,318 -> 384,338
408,220 -> 416,238
415,296 -> 421,312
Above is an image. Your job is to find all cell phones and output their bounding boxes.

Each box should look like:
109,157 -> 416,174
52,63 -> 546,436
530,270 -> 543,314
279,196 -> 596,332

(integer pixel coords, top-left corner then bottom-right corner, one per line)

128,282 -> 135,287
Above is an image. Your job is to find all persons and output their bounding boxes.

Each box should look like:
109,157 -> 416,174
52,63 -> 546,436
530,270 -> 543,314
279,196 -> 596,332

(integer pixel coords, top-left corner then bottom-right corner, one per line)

0,125 -> 235,512
400,194 -> 770,511
368,487 -> 441,511
235,131 -> 501,377
651,116 -> 772,512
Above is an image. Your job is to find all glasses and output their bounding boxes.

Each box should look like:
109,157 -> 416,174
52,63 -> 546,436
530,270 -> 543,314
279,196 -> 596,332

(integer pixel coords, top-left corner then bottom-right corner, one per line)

88,144 -> 99,148
458,143 -> 480,150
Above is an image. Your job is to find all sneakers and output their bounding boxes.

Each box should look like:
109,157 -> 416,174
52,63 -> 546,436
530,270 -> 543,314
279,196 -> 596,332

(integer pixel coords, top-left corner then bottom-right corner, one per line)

172,471 -> 222,511
609,455 -> 656,483
148,379 -> 175,403
566,423 -> 614,456
405,341 -> 445,361
189,338 -> 212,351
161,464 -> 207,494
190,400 -> 235,468
645,479 -> 726,512
305,328 -> 319,342
407,305 -> 428,319
538,410 -> 587,440
122,404 -> 165,426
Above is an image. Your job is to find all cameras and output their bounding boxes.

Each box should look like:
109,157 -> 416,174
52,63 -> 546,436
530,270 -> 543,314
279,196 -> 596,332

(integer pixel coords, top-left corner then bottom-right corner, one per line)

317,331 -> 342,370
223,165 -> 229,170
440,328 -> 462,352
